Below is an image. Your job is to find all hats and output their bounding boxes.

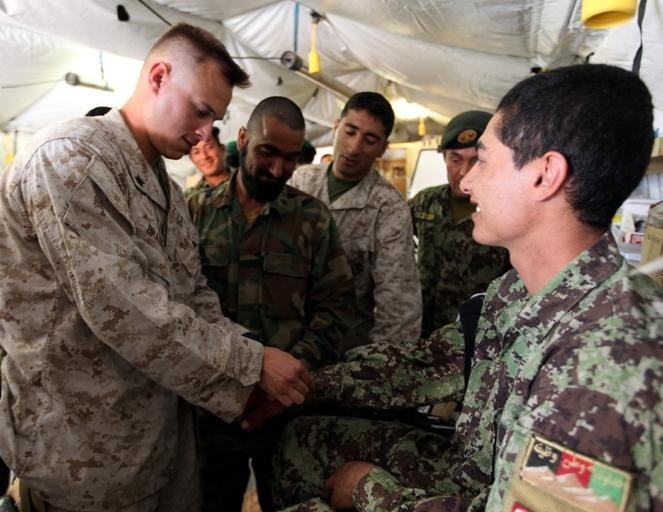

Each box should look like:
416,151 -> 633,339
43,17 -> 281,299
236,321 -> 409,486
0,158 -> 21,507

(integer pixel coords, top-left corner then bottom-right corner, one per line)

298,139 -> 316,162
226,141 -> 239,158
440,111 -> 493,150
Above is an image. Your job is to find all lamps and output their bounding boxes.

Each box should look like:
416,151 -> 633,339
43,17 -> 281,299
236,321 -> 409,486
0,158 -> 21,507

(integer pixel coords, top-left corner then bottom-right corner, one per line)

417,116 -> 427,137
581,0 -> 637,29
306,9 -> 322,75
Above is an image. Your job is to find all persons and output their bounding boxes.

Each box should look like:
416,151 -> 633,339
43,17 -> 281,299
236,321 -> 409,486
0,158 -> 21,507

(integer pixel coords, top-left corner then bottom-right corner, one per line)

1,23 -> 315,512
182,91 -> 423,511
406,110 -> 516,341
248,64 -> 662,512
